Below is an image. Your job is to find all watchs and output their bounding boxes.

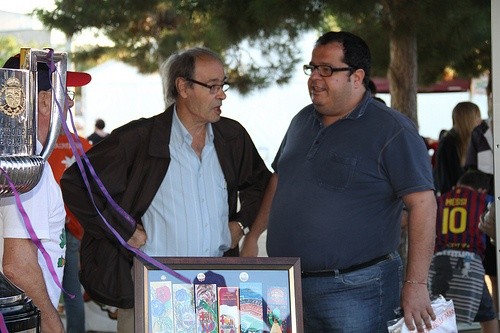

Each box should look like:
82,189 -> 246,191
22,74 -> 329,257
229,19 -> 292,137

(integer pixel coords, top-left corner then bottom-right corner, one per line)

237,221 -> 250,234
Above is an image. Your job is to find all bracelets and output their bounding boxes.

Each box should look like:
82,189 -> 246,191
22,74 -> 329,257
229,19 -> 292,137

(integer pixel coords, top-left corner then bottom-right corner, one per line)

403,280 -> 428,286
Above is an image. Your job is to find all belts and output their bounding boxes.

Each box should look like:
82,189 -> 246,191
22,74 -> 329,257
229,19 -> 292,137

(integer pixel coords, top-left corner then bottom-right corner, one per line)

301,251 -> 394,277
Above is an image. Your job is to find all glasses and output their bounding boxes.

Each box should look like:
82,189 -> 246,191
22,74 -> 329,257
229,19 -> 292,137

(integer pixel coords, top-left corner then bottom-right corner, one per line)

186,78 -> 230,94
303,65 -> 353,77
49,91 -> 76,101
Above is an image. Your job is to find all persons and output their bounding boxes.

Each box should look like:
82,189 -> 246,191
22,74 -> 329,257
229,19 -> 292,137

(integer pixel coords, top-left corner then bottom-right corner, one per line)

0,53 -> 91,333
48,126 -> 92,333
237,31 -> 437,333
86,119 -> 110,144
366,77 -> 500,333
63,44 -> 274,333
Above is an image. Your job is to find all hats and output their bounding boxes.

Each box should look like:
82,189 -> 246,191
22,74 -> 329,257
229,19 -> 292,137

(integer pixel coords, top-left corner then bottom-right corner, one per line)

2,53 -> 92,90
